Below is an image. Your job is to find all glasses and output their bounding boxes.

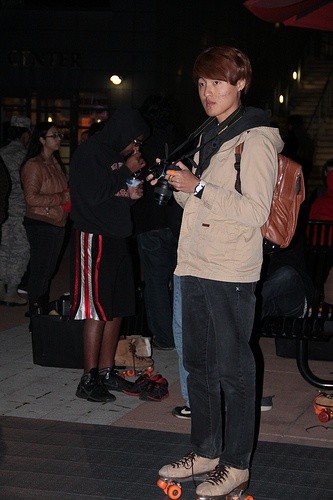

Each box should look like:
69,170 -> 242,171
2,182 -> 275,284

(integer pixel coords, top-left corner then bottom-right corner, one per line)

46,134 -> 64,139
133,139 -> 145,145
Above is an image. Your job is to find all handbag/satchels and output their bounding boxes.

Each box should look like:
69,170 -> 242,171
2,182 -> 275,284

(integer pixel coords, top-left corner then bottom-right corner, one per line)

235,142 -> 305,249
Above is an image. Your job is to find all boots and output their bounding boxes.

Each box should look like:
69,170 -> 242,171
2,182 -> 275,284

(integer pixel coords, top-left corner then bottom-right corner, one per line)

0,281 -> 27,306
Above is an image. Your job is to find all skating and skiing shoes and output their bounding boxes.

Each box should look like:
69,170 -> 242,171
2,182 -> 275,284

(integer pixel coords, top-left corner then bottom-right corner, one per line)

196,463 -> 254,500
312,392 -> 333,423
157,452 -> 220,500
114,336 -> 155,377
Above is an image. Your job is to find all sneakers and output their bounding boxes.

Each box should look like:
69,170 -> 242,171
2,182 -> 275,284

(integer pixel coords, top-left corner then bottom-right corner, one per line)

76,368 -> 116,402
99,367 -> 130,391
172,406 -> 192,420
140,378 -> 169,402
123,374 -> 162,396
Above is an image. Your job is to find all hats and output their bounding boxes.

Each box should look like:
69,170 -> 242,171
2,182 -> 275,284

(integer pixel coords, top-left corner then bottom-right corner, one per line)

10,114 -> 32,132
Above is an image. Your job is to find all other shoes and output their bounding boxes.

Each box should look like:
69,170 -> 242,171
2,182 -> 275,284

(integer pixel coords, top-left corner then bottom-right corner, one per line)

300,299 -> 333,321
260,395 -> 275,412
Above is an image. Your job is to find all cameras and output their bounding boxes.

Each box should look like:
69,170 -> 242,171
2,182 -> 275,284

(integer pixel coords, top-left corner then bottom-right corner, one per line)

148,159 -> 182,207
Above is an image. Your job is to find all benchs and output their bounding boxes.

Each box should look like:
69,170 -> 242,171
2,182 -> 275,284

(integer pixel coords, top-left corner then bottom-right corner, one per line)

129,214 -> 333,390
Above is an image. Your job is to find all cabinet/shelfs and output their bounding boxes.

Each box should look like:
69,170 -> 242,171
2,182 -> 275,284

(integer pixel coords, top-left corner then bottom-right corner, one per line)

0,89 -> 110,164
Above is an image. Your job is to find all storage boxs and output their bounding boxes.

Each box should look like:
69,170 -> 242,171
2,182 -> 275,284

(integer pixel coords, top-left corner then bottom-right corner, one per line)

24,299 -> 85,369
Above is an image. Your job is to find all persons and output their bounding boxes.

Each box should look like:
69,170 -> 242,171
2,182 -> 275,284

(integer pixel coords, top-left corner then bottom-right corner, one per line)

68,105 -> 143,403
171,203 -> 314,421
271,114 -> 333,250
19,122 -> 71,315
0,155 -> 13,225
1,114 -> 32,307
132,93 -> 187,350
146,45 -> 284,500
